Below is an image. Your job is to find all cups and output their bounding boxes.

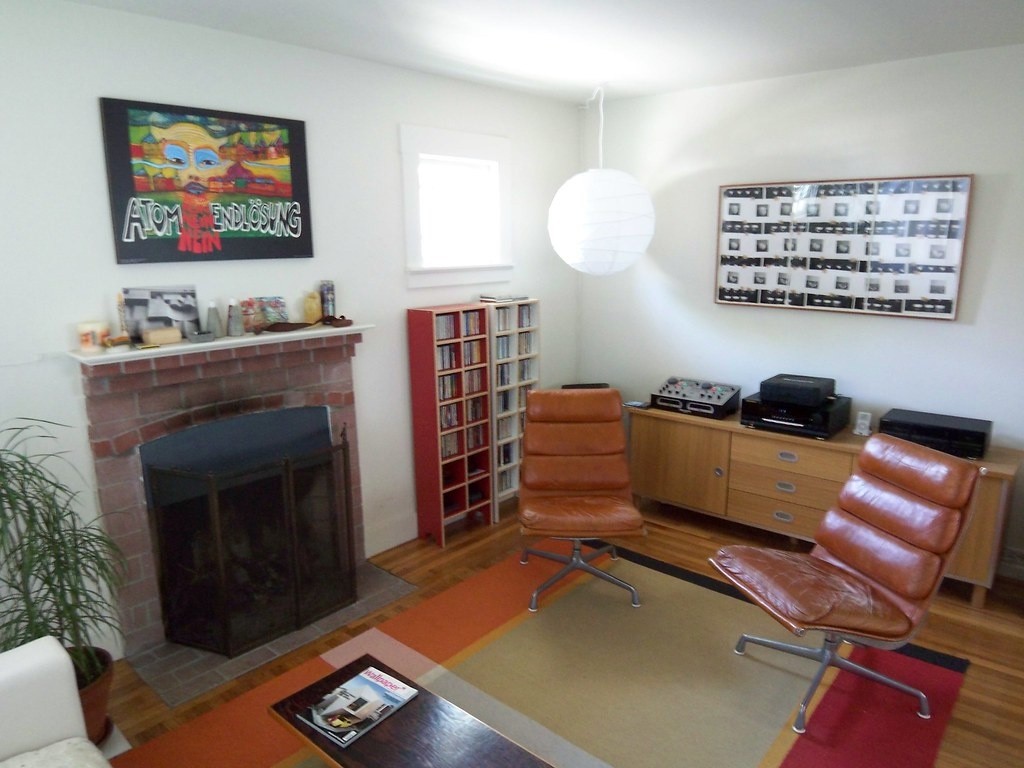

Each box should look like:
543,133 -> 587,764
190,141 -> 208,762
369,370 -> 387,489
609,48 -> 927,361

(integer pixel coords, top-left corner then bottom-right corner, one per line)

78,322 -> 109,352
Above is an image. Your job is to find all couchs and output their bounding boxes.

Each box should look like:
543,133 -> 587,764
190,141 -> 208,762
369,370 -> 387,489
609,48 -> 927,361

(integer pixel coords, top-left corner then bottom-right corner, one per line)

0,635 -> 112,768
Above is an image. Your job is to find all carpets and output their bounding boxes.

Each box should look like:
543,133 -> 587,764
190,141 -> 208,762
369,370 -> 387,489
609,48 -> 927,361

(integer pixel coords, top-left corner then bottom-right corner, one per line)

108,536 -> 968,768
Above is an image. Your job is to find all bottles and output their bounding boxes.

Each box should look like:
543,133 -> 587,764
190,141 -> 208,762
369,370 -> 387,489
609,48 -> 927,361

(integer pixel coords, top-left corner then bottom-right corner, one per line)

320,280 -> 335,317
206,300 -> 223,338
227,298 -> 244,336
304,291 -> 322,323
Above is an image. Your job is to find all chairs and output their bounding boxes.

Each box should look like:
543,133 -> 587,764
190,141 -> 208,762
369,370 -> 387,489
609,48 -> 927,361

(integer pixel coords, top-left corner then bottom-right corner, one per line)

707,433 -> 987,734
517,389 -> 647,613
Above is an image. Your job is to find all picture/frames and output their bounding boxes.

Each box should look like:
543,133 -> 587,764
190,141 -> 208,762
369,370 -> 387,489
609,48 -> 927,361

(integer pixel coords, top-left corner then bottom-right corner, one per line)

99,96 -> 315,265
714,173 -> 973,322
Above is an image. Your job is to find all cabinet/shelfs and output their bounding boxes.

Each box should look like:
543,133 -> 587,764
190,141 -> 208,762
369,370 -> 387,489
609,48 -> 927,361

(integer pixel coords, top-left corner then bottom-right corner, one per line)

624,405 -> 1023,606
407,297 -> 542,549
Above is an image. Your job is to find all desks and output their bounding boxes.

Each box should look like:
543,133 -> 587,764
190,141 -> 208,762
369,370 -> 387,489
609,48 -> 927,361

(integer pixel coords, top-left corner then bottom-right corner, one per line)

266,654 -> 556,768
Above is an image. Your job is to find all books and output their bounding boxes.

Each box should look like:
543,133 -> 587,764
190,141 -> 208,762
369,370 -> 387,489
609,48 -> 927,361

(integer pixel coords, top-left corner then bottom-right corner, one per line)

295,665 -> 419,748
435,304 -> 532,492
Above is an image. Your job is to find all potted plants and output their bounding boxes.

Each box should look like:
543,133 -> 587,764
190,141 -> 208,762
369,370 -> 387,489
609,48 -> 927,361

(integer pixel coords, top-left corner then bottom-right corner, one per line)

0,416 -> 142,748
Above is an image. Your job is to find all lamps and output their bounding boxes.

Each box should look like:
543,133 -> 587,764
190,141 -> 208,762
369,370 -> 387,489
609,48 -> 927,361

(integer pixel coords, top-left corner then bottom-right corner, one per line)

545,86 -> 659,278
